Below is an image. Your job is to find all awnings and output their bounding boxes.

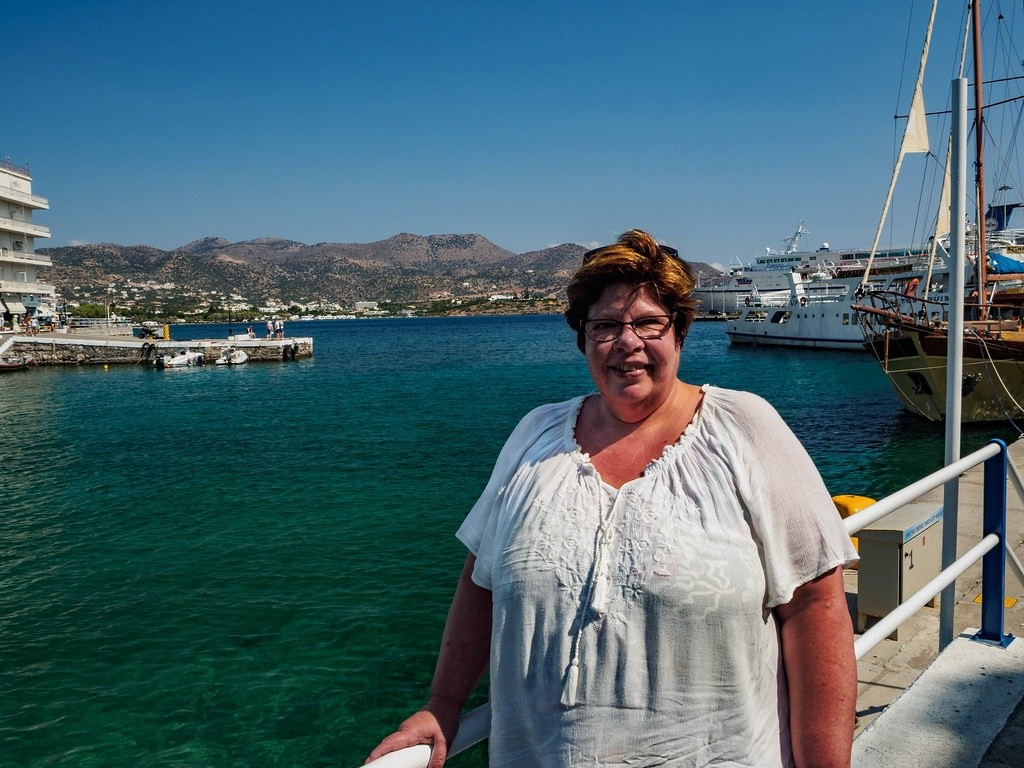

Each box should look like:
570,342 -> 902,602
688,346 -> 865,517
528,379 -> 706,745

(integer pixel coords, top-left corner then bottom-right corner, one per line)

3,295 -> 27,314
0,301 -> 6,312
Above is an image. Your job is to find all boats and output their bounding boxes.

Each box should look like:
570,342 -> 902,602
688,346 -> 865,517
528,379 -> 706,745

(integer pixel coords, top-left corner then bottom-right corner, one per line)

215,342 -> 249,366
151,347 -> 206,369
0,348 -> 35,372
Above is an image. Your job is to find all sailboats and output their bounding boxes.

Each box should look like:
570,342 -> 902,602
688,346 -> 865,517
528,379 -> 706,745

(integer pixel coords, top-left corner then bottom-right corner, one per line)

682,0 -> 1024,431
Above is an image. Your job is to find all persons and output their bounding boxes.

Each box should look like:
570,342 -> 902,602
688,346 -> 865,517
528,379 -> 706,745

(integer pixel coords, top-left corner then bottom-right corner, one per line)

111,312 -> 118,326
12,315 -> 40,337
247,325 -> 256,339
266,318 -> 284,341
0,315 -> 4,331
364,231 -> 857,768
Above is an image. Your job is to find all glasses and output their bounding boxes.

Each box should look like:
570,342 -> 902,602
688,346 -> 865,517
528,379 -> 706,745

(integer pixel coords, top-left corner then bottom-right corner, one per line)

583,243 -> 679,259
580,312 -> 678,342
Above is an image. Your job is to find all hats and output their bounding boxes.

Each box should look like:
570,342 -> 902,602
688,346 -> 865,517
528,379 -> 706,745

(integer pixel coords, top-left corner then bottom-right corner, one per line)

14,315 -> 19,317
267,321 -> 272,324
